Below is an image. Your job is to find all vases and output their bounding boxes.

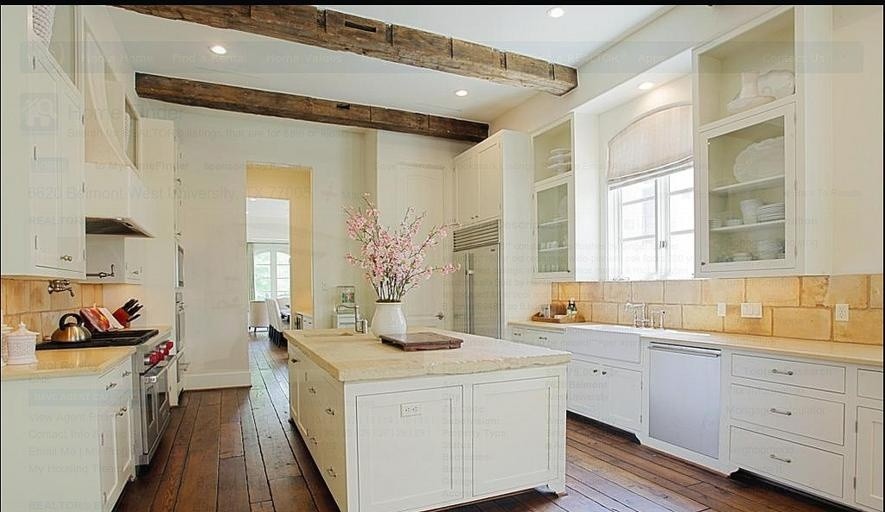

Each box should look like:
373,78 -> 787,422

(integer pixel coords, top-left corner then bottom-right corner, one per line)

372,301 -> 406,341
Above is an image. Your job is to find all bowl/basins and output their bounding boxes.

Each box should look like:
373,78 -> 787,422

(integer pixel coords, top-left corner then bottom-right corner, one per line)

537,240 -> 569,273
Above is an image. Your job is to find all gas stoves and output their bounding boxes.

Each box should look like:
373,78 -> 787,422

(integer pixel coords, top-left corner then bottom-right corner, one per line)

36,329 -> 159,350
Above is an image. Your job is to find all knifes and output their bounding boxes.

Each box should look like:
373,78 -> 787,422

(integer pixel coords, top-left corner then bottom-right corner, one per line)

122,298 -> 143,322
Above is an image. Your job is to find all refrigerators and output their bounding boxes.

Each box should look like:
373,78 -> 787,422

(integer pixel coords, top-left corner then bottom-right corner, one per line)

452,242 -> 501,338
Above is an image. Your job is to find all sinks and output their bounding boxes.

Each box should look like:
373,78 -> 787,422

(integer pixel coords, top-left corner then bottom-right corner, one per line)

583,324 -> 708,336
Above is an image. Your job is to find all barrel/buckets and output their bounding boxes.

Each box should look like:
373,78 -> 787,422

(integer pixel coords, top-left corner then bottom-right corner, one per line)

335,286 -> 355,313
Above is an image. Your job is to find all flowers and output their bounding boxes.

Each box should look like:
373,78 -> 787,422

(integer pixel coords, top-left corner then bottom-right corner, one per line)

338,190 -> 461,300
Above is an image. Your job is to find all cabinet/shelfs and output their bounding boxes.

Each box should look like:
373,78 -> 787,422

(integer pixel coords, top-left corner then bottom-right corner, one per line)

508,326 -> 562,354
452,131 -> 532,232
140,115 -> 188,408
530,115 -> 599,278
1,39 -> 89,283
567,360 -> 642,430
1,358 -> 131,512
848,365 -> 885,512
691,5 -> 834,279
721,349 -> 848,507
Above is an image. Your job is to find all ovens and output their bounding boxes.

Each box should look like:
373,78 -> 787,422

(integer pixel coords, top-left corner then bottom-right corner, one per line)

132,357 -> 177,466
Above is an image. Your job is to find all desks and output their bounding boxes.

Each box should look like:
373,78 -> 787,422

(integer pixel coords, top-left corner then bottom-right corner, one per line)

282,325 -> 573,511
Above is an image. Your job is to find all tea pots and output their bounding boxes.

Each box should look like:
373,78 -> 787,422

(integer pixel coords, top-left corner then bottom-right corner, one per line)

51,312 -> 92,343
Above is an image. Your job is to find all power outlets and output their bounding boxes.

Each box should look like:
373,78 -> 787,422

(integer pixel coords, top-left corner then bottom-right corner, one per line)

835,302 -> 849,322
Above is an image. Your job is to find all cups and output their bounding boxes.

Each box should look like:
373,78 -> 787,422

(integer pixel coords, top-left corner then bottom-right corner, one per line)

5,322 -> 39,365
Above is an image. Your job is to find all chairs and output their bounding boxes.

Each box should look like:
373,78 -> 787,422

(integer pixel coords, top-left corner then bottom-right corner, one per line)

250,296 -> 290,349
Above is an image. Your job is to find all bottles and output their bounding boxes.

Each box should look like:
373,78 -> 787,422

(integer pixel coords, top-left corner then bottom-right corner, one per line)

565,299 -> 577,322
727,72 -> 777,114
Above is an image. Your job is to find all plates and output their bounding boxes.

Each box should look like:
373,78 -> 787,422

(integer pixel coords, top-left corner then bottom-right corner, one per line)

709,198 -> 787,261
546,147 -> 572,173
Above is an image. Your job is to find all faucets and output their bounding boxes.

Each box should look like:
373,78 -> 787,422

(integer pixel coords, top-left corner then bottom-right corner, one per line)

625,302 -> 648,327
659,310 -> 666,330
